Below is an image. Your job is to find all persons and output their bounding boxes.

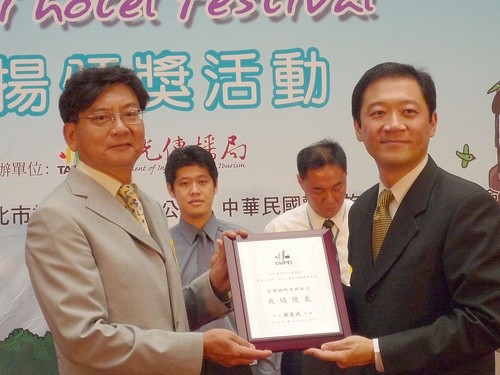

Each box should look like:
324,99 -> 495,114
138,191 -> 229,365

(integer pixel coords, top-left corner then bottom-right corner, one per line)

303,62 -> 499,374
164,145 -> 276,375
255,137 -> 354,375
24,65 -> 272,375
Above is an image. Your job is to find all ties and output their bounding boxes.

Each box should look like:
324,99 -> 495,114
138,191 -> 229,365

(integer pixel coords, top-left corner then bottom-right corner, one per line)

371,189 -> 394,264
195,229 -> 211,278
322,220 -> 342,283
118,184 -> 153,240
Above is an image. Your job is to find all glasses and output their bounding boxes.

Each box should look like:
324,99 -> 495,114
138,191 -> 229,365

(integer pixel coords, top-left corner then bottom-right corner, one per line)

69,108 -> 147,124
